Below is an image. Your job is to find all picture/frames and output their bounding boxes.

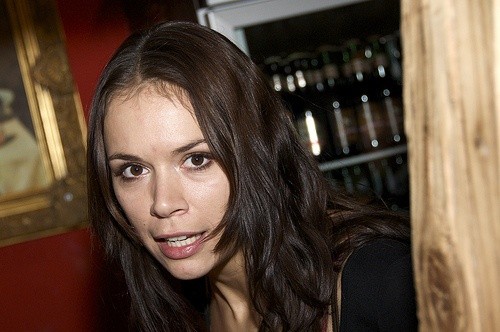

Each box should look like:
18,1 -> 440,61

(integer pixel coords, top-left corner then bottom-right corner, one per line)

0,0 -> 95,250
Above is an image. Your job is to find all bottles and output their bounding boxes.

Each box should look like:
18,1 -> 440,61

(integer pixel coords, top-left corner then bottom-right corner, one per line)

269,32 -> 408,212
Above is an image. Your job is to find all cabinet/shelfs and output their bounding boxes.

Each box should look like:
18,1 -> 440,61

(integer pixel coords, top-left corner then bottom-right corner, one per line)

198,3 -> 412,215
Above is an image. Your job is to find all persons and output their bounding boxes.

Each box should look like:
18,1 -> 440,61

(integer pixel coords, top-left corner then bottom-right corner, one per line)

82,22 -> 424,332
